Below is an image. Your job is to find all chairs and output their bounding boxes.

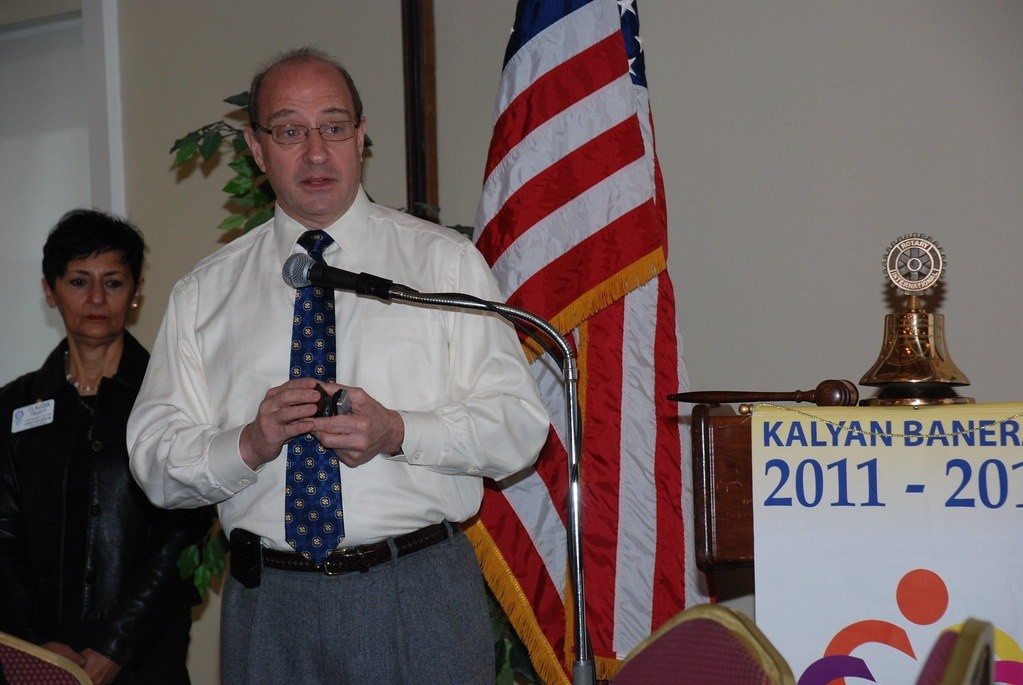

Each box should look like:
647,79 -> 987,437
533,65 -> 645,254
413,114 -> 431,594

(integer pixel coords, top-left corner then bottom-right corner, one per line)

0,630 -> 96,685
913,617 -> 993,685
601,602 -> 795,684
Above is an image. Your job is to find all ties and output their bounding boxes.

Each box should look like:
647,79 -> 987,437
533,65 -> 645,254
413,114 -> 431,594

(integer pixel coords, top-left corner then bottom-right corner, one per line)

284,230 -> 346,569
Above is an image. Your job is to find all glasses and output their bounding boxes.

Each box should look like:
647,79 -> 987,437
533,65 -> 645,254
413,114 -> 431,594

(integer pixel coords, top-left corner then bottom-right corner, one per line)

256,116 -> 363,144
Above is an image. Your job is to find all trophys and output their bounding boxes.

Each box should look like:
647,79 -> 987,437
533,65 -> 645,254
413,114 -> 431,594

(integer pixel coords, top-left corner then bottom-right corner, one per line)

858,233 -> 977,406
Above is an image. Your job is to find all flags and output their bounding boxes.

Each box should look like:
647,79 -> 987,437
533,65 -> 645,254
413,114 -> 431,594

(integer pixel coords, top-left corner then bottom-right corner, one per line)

455,0 -> 718,685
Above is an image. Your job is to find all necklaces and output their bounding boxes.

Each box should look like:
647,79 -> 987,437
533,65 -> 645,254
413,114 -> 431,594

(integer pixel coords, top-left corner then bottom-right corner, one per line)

65,351 -> 98,390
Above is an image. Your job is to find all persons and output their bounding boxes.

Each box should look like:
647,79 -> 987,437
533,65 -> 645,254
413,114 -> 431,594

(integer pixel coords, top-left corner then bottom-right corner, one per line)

127,51 -> 551,685
1,210 -> 217,684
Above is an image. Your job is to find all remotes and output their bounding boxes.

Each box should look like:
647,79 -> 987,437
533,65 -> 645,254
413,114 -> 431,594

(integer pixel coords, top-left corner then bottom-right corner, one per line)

330,389 -> 351,416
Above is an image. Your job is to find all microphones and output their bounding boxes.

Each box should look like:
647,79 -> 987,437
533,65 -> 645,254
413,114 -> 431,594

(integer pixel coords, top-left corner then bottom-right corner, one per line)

281,253 -> 420,300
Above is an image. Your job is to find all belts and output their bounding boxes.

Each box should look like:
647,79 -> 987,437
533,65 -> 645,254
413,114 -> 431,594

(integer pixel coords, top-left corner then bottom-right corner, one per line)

262,519 -> 459,575
